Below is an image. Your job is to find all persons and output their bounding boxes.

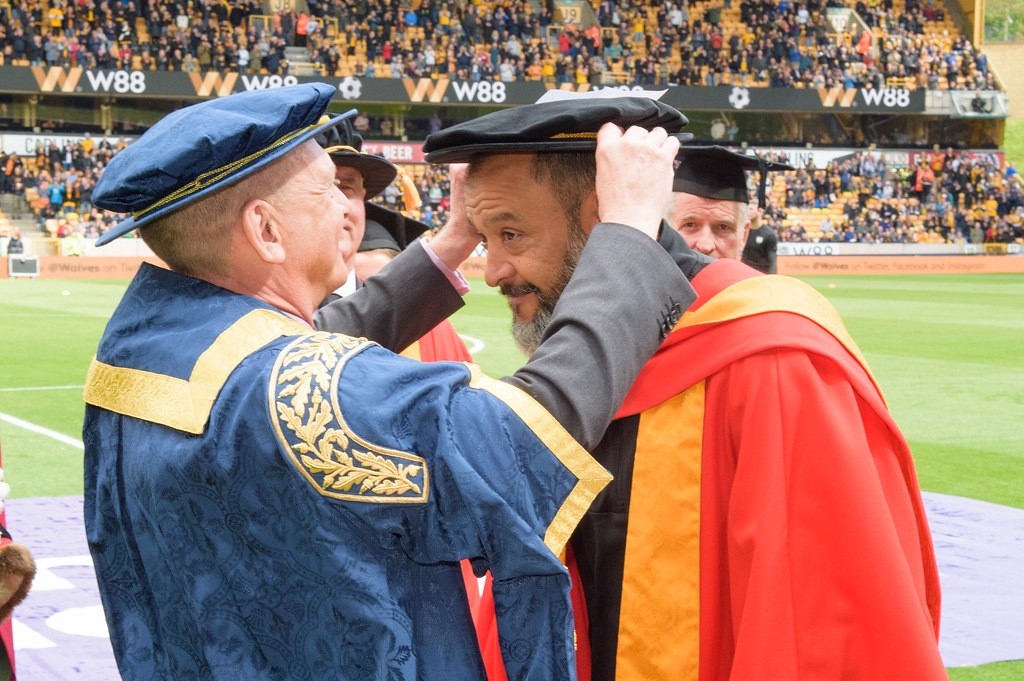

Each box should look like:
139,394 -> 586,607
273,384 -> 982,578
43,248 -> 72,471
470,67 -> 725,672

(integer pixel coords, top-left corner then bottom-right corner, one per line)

315,113 -> 369,320
665,152 -> 749,265
426,91 -> 948,681
77,80 -> 696,681
742,199 -> 778,276
7,232 -> 23,254
0,0 -> 1024,242
351,218 -> 474,364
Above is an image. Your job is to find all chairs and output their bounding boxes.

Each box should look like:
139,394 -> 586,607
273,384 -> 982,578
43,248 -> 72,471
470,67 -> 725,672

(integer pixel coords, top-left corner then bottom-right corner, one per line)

23,153 -> 1024,244
1,1 -> 1001,89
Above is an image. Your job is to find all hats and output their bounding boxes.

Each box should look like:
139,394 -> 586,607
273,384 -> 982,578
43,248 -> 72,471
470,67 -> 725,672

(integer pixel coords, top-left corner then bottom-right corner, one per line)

672,144 -> 796,205
91,83 -> 359,246
312,110 -> 396,200
355,201 -> 431,253
422,97 -> 694,162
